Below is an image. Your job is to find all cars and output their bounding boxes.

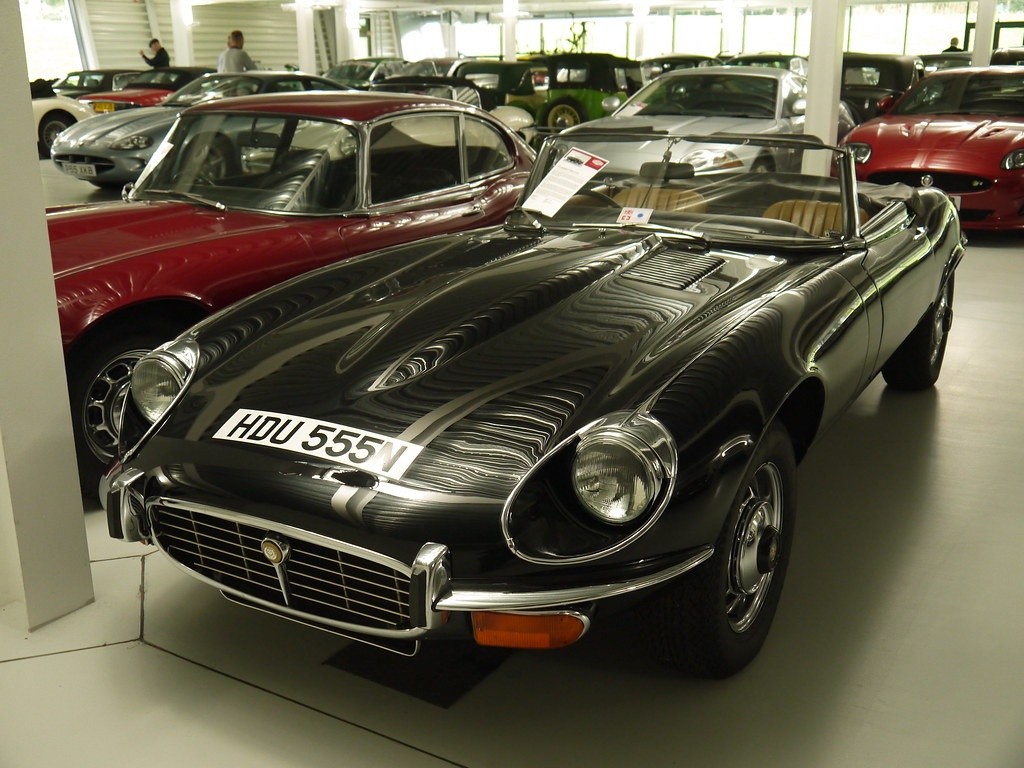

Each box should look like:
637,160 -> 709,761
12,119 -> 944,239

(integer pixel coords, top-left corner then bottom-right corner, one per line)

44,84 -> 541,474
97,128 -> 970,689
30,52 -> 1024,237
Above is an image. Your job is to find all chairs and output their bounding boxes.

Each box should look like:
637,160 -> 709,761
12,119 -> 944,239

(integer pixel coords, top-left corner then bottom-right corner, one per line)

763,199 -> 868,236
609,187 -> 709,214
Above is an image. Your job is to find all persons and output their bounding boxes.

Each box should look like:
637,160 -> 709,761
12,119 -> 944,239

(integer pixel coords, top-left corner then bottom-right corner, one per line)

140,39 -> 171,83
937,37 -> 969,70
218,31 -> 257,96
